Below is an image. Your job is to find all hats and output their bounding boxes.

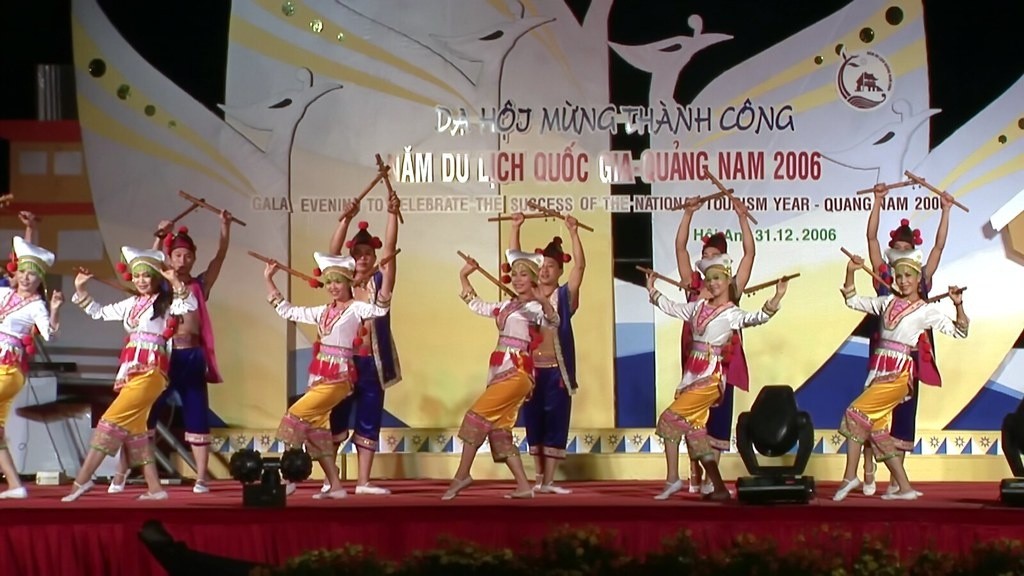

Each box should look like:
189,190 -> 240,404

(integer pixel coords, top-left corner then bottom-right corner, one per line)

7,236 -> 56,279
505,236 -> 571,277
695,232 -> 732,278
884,218 -> 923,273
120,226 -> 197,273
313,221 -> 382,280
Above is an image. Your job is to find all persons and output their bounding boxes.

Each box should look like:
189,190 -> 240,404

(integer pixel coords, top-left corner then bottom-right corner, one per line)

0,211 -> 66,499
319,189 -> 400,497
861,183 -> 956,500
674,195 -> 756,495
504,213 -> 588,495
440,249 -> 561,500
59,246 -> 180,502
644,252 -> 789,500
831,250 -> 970,502
107,208 -> 233,495
264,252 -> 390,499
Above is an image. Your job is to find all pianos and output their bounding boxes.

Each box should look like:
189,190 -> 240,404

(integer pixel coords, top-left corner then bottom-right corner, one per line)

26,361 -> 79,379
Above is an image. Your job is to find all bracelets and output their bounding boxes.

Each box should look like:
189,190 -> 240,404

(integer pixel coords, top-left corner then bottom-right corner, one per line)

953,300 -> 963,306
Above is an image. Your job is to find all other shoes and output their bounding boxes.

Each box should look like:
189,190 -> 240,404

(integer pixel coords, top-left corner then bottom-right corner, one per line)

711,491 -> 731,500
137,490 -> 168,500
886,486 -> 924,495
0,487 -> 28,498
193,479 -> 209,494
881,489 -> 916,501
504,489 -> 534,498
286,482 -> 296,495
441,476 -> 474,500
689,481 -> 714,494
355,482 -> 391,494
108,472 -> 126,493
321,467 -> 340,493
312,490 -> 347,499
654,479 -> 683,499
863,463 -> 877,496
533,481 -> 570,494
61,479 -> 95,502
833,476 -> 860,502
